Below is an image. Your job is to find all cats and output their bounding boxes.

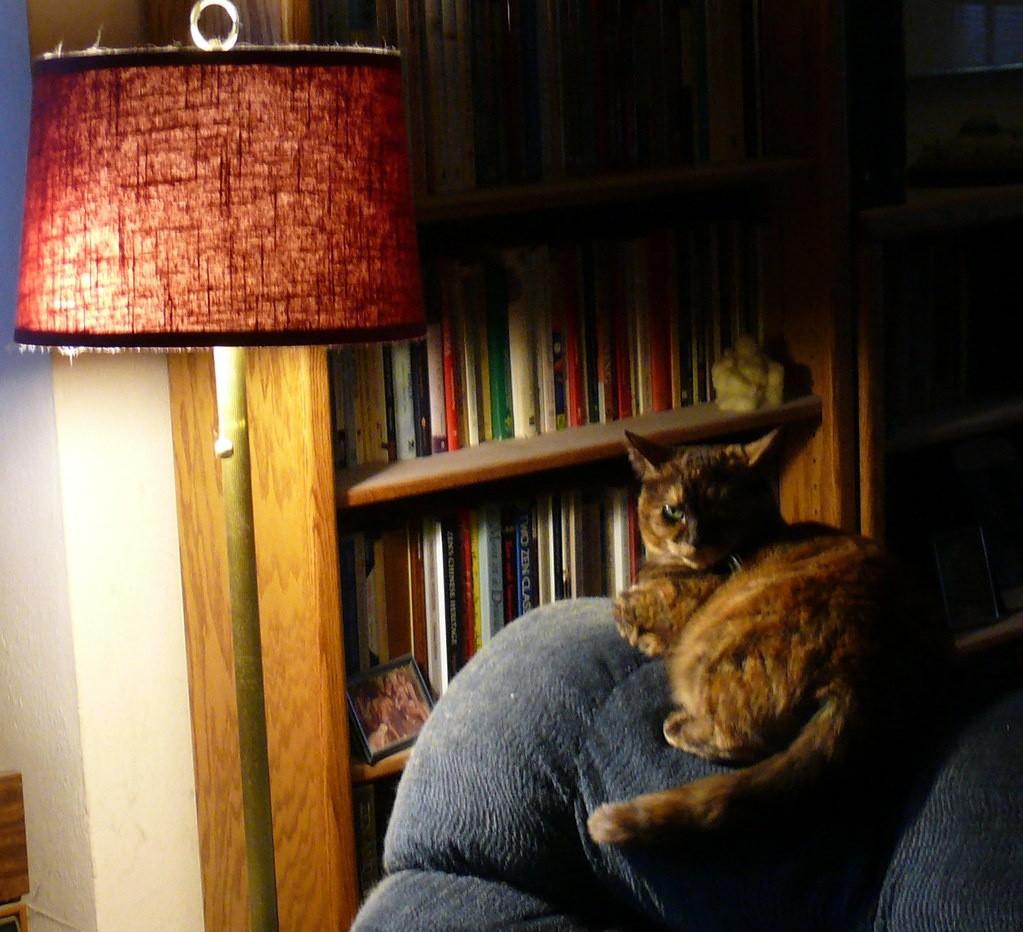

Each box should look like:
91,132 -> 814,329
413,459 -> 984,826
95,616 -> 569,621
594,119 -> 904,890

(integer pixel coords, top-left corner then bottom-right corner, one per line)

587,427 -> 919,846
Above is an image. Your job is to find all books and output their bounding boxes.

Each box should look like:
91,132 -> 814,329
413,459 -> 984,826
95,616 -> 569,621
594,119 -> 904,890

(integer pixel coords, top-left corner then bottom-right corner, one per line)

327,219 -> 744,468
888,258 -> 968,414
345,0 -> 747,195
333,482 -> 645,700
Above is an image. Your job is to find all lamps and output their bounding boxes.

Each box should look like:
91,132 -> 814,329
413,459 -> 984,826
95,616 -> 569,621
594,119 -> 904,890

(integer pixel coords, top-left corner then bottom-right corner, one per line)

14,1 -> 426,932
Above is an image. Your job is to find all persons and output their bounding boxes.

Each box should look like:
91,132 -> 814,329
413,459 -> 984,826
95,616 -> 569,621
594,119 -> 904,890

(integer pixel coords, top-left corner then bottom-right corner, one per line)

368,671 -> 430,740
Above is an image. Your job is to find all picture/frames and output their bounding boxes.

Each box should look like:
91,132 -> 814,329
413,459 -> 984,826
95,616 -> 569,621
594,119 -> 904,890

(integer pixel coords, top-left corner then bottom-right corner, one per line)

346,654 -> 435,767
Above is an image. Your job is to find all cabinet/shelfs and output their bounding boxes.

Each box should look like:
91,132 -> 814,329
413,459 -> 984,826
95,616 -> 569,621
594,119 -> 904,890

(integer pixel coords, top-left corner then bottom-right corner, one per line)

141,0 -> 1022,932
